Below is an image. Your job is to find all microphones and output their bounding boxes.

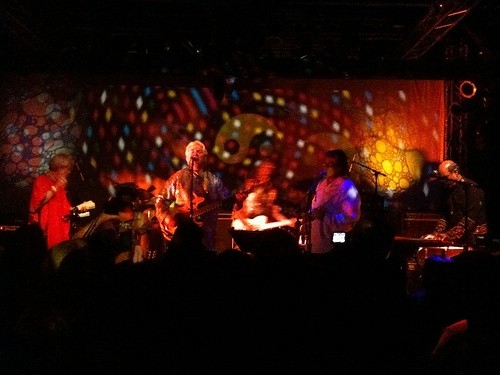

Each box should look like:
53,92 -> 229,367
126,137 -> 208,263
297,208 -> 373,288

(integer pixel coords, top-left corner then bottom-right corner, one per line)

317,170 -> 327,175
431,172 -> 446,180
191,156 -> 199,162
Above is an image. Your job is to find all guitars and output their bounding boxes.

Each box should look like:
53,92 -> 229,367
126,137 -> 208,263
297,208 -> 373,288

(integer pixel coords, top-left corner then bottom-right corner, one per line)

157,179 -> 271,242
230,203 -> 332,237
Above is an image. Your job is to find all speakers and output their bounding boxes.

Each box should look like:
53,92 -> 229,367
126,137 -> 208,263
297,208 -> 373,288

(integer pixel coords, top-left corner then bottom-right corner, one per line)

451,80 -> 482,115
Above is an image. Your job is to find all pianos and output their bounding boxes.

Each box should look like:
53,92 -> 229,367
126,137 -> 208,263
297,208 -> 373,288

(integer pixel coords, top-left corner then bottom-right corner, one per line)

389,231 -> 457,253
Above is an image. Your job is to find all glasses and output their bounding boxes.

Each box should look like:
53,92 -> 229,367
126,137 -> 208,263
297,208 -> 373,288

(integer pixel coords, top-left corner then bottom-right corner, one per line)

321,161 -> 338,168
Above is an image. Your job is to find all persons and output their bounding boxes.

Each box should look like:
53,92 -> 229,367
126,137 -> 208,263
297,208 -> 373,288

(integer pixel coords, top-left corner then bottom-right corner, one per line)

299,149 -> 362,255
421,160 -> 487,244
29,154 -> 87,248
231,161 -> 296,230
155,141 -> 248,251
